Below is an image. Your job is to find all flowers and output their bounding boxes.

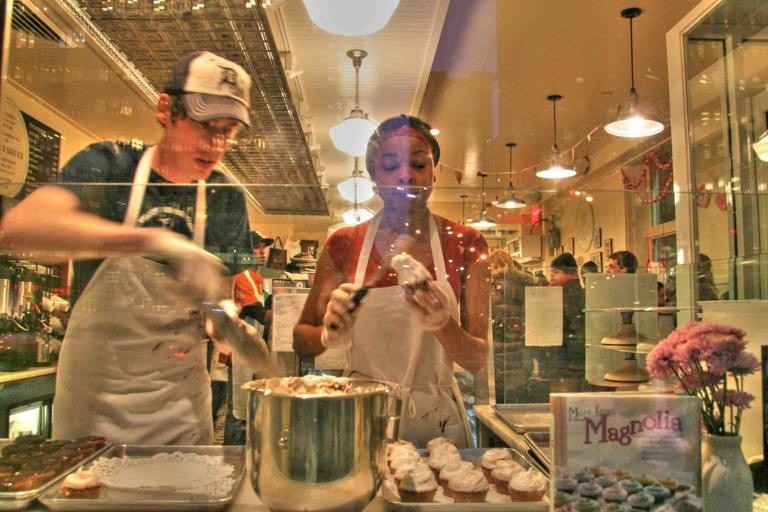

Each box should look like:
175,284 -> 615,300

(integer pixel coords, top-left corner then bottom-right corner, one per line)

646,321 -> 761,435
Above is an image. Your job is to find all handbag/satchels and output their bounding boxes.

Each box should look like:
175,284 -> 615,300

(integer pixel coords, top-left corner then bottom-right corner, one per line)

267,235 -> 289,270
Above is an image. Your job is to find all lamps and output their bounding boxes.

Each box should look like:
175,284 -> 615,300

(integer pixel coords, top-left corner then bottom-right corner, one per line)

470,173 -> 496,227
330,50 -> 379,157
337,156 -> 374,203
536,95 -> 576,179
343,203 -> 374,225
604,6 -> 665,138
495,143 -> 526,208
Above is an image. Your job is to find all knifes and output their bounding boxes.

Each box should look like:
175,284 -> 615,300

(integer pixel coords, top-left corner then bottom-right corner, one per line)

327,230 -> 415,333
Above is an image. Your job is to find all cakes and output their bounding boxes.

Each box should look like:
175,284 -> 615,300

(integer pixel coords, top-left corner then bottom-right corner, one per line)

384,436 -> 549,501
389,250 -> 433,293
553,466 -> 680,510
0,434 -> 107,497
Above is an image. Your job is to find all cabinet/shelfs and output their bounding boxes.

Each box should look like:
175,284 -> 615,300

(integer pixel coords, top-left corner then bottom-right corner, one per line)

583,306 -> 697,391
507,235 -> 541,262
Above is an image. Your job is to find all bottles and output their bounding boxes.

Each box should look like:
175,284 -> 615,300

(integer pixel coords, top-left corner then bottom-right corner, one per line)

0,276 -> 50,373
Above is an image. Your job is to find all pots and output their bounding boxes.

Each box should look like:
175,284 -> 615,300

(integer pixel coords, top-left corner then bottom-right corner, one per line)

238,376 -> 391,511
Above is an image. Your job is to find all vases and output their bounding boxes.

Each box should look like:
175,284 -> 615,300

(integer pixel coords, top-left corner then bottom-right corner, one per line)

702,435 -> 754,512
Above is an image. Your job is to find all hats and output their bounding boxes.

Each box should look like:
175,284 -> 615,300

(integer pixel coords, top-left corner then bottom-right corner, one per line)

251,230 -> 275,247
551,252 -> 578,272
163,48 -> 253,130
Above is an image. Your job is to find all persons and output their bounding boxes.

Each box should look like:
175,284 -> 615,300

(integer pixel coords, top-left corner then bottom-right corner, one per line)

0,50 -> 260,446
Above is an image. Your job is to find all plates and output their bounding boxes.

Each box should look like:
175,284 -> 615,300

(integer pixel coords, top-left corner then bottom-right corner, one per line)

90,453 -> 235,502
288,256 -> 318,274
